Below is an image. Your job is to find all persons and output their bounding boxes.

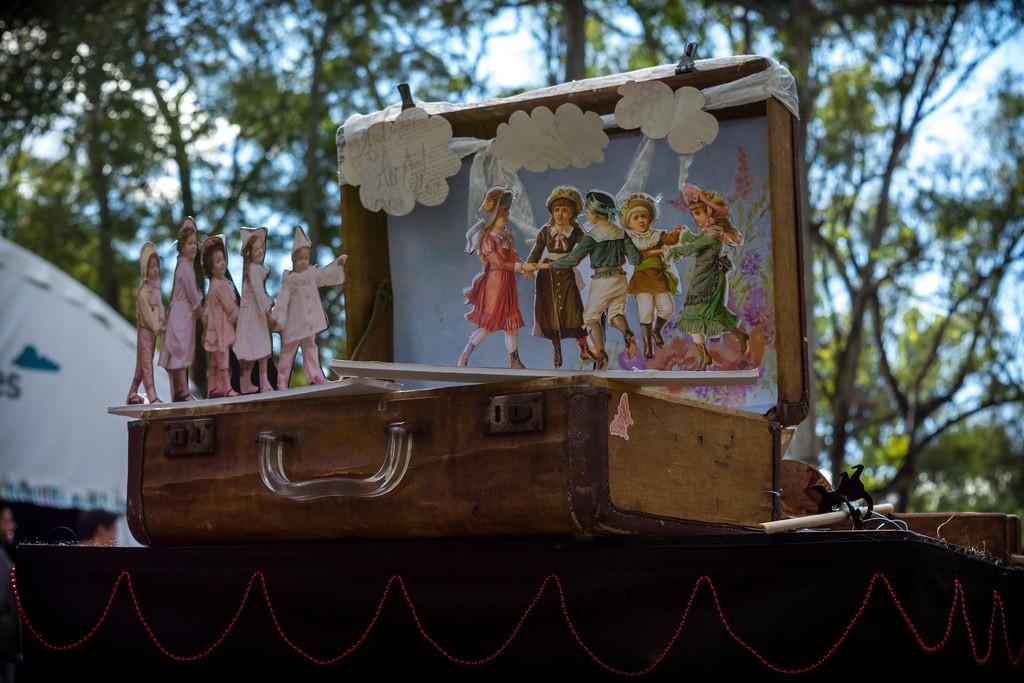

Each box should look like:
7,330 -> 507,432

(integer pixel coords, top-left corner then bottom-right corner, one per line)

199,232 -> 242,398
270,224 -> 348,392
616,190 -> 690,360
457,186 -> 535,370
642,188 -> 752,373
122,241 -> 168,406
522,184 -> 598,369
7,486 -> 120,549
527,190 -> 649,370
156,215 -> 204,402
231,225 -> 277,395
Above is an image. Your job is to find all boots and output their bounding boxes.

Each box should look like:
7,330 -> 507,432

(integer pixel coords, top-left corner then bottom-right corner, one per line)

652,327 -> 665,348
643,335 -> 655,362
457,342 -> 475,367
731,327 -> 752,356
240,376 -> 259,395
510,349 -> 526,369
695,343 -> 714,371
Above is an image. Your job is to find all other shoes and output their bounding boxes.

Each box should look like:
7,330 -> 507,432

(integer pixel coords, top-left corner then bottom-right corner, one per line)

128,394 -> 144,404
224,388 -> 242,396
593,352 -> 609,371
174,390 -> 198,401
150,397 -> 165,403
579,345 -> 598,361
624,331 -> 637,361
553,345 -> 563,369
209,387 -> 224,399
260,375 -> 275,393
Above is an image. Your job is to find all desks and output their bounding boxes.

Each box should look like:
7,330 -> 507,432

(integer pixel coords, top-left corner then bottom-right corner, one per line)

9,522 -> 1019,683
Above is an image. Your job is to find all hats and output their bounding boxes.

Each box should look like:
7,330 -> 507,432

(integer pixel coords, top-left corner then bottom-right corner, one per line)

478,186 -> 513,230
239,226 -> 268,257
200,236 -> 222,266
621,191 -> 659,231
139,242 -> 161,279
545,184 -> 585,215
291,226 -> 312,255
681,183 -> 730,218
585,190 -> 624,223
170,216 -> 197,251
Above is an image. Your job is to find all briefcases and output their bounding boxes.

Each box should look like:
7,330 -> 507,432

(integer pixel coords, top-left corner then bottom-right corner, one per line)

128,53 -> 811,531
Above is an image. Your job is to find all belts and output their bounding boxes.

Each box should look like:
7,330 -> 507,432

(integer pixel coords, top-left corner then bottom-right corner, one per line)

594,265 -> 623,273
